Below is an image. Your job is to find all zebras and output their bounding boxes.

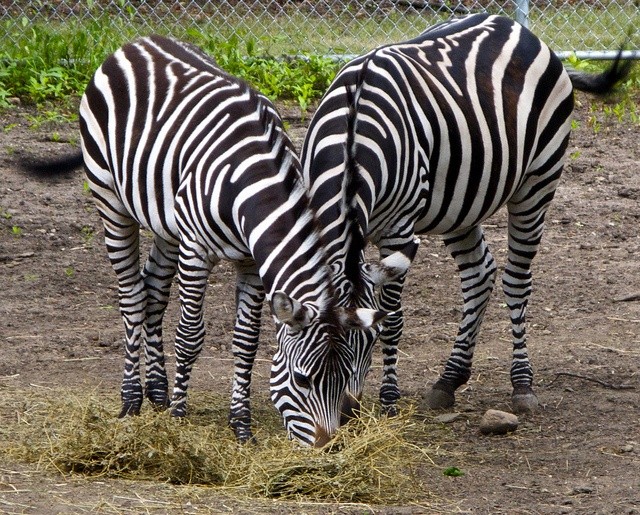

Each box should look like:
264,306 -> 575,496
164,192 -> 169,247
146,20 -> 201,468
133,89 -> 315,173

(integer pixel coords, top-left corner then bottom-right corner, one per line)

78,34 -> 408,453
300,13 -> 640,428
